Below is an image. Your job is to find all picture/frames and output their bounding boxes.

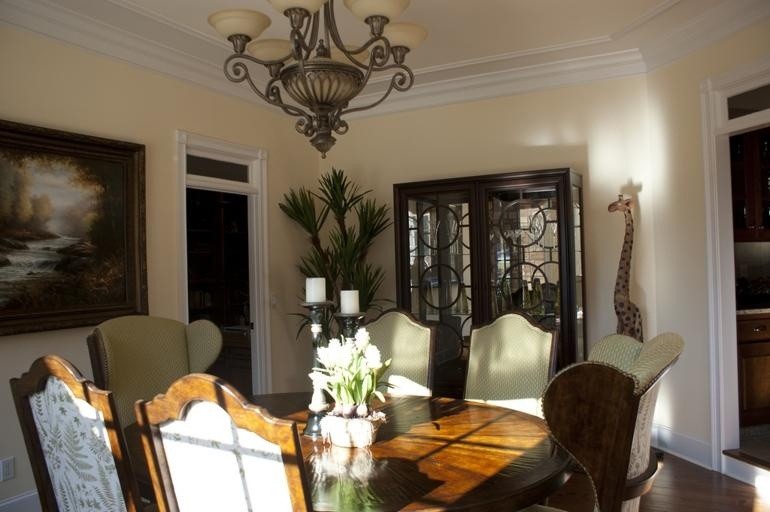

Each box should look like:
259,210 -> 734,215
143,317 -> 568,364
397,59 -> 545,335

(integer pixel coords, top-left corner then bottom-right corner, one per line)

0,120 -> 149,337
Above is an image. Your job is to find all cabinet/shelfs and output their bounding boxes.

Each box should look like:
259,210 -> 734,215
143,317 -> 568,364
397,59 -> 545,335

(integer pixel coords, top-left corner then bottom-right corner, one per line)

212,329 -> 252,398
394,168 -> 586,400
730,127 -> 770,242
736,309 -> 770,429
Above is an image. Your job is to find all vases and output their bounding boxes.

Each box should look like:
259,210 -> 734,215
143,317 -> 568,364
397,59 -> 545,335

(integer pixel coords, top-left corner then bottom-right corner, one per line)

321,416 -> 380,447
321,447 -> 372,483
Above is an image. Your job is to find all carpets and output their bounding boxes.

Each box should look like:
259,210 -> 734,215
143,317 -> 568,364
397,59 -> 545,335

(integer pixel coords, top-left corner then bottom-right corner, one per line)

738,426 -> 769,466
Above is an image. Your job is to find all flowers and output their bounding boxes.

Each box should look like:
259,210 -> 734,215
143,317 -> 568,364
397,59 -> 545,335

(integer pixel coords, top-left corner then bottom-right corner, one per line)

308,328 -> 395,416
314,481 -> 383,508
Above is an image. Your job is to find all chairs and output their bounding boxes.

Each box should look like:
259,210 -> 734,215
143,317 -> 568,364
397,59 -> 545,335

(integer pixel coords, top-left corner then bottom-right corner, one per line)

8,356 -> 158,511
134,373 -> 313,510
526,334 -> 683,512
355,309 -> 436,396
462,307 -> 557,421
86,315 -> 224,512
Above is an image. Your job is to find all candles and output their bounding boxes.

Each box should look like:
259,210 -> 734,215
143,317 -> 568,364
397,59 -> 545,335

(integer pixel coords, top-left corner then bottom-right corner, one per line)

341,290 -> 366,317
305,277 -> 326,302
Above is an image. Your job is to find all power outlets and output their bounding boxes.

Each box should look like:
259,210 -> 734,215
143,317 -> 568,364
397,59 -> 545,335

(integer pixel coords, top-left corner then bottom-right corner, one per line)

1,457 -> 15,482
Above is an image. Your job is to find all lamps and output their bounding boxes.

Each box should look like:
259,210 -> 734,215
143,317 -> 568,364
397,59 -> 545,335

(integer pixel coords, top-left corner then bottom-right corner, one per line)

208,1 -> 426,158
